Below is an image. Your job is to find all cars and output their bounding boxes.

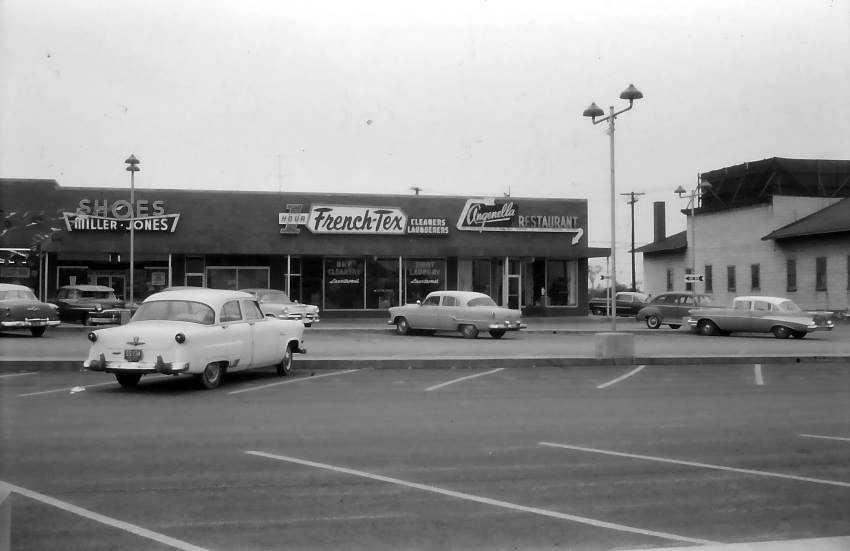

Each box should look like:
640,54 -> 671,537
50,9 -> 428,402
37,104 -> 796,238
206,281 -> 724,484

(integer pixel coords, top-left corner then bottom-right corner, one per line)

387,290 -> 528,340
46,284 -> 140,326
82,285 -> 308,390
0,281 -> 61,338
233,287 -> 320,328
636,291 -> 716,330
588,291 -> 651,317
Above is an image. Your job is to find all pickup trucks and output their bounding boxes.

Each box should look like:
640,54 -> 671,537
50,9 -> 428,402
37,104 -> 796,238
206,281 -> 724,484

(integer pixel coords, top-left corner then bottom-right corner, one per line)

687,295 -> 819,339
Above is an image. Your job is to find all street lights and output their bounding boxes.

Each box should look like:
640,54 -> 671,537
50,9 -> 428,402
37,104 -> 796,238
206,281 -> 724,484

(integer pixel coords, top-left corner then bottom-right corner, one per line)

124,154 -> 141,307
582,82 -> 645,334
674,180 -> 713,295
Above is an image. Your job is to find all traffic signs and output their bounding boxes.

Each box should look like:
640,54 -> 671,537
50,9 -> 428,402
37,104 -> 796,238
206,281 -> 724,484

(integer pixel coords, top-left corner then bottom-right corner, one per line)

684,273 -> 705,284
